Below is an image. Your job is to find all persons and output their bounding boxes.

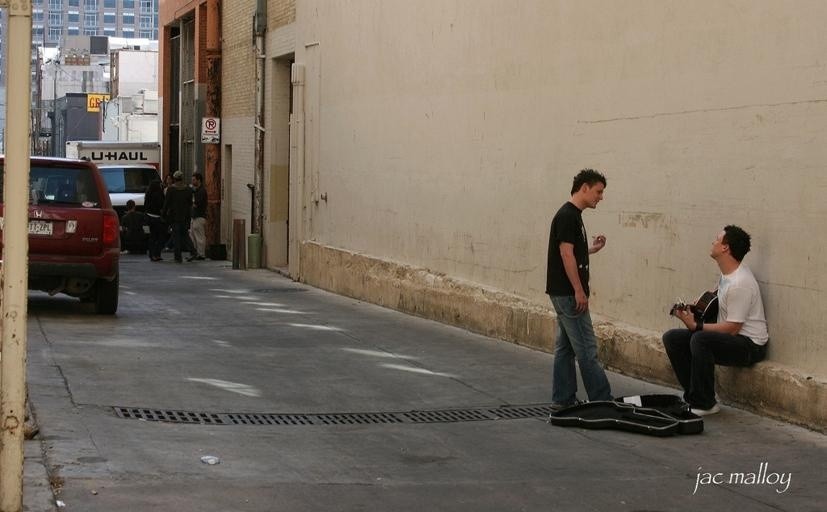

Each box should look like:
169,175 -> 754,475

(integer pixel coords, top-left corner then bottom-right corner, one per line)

122,171 -> 207,263
545,168 -> 615,409
663,225 -> 770,416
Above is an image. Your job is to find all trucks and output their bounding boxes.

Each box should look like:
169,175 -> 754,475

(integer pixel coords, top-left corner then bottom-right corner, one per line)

65,141 -> 161,254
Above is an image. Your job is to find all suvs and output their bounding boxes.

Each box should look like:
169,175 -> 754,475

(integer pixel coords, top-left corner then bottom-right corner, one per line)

0,153 -> 121,314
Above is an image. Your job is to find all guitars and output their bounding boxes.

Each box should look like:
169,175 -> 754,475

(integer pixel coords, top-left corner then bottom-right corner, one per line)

670,290 -> 718,324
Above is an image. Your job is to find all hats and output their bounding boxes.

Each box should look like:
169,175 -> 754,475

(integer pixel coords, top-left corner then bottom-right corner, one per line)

173,170 -> 185,179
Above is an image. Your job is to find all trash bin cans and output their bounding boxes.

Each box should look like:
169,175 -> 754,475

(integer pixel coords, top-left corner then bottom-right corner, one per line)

248,234 -> 261,269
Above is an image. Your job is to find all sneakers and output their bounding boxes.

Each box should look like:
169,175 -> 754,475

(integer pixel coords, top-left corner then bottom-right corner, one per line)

150,255 -> 163,261
688,392 -> 720,416
186,254 -> 205,262
551,400 -> 588,411
